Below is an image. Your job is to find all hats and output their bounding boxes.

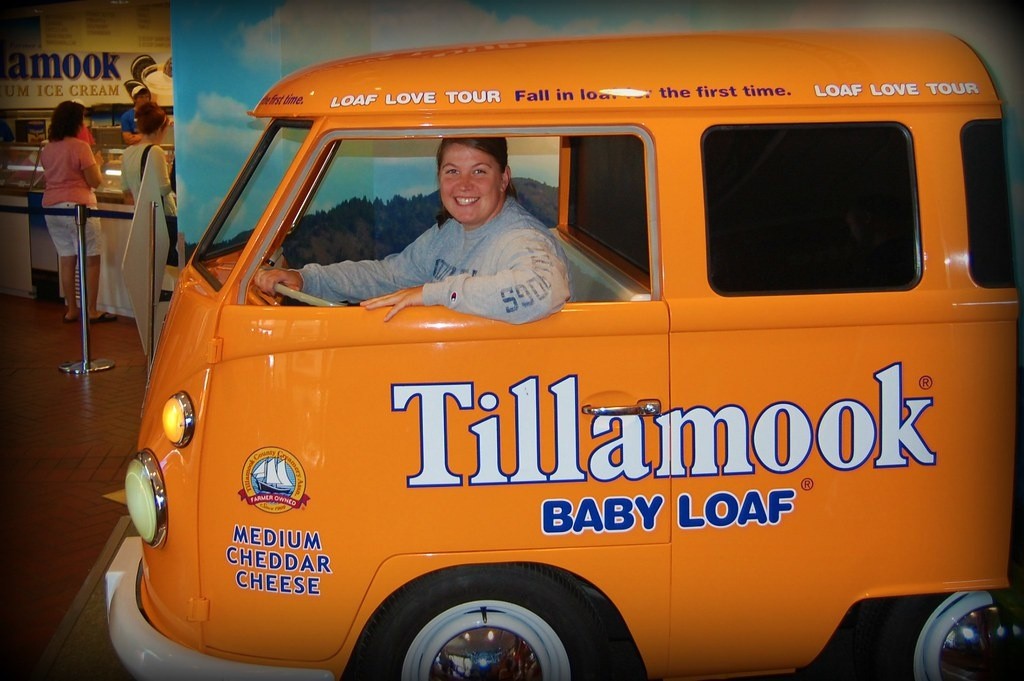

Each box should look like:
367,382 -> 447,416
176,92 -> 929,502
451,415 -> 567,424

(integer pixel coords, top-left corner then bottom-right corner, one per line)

131,85 -> 146,97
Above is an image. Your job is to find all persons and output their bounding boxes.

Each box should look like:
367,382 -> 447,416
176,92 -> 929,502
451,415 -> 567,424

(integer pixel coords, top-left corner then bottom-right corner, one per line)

122,86 -> 178,301
41,100 -> 115,322
252,137 -> 570,324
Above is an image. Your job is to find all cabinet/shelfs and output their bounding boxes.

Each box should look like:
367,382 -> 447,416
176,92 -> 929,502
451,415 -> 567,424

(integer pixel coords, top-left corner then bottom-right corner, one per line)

91,143 -> 125,201
0,141 -> 45,193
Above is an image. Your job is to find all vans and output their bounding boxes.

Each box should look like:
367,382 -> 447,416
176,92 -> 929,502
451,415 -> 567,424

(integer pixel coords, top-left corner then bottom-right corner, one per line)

105,28 -> 1024,681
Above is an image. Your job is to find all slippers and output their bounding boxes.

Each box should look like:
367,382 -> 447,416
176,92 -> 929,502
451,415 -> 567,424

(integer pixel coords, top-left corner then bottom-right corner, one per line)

63,312 -> 78,322
89,312 -> 117,322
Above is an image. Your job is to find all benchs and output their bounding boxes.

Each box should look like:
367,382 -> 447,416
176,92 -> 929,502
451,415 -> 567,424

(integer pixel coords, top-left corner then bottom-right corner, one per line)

544,227 -> 650,303
